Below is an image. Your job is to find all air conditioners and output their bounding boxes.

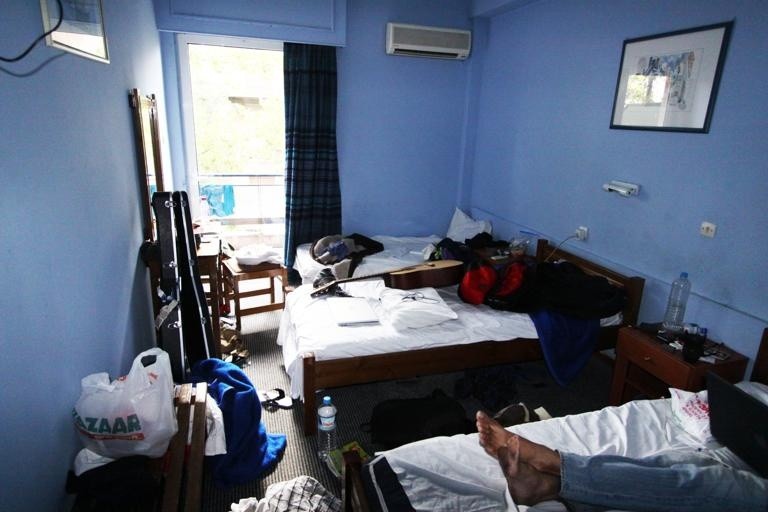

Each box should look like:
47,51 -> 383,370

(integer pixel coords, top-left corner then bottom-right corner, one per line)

385,23 -> 472,59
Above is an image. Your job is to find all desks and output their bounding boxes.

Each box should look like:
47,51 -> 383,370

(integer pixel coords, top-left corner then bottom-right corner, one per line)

192,219 -> 225,360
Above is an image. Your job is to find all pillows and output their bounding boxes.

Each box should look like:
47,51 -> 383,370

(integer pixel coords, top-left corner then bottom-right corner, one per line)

446,207 -> 491,243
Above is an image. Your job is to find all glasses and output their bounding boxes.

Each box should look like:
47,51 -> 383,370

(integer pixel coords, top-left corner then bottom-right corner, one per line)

402,292 -> 439,303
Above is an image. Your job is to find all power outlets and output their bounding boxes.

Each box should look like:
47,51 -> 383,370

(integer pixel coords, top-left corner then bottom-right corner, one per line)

579,226 -> 588,240
699,221 -> 718,238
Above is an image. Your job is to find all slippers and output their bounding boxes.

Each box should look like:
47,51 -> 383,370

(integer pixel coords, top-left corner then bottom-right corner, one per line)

257,388 -> 292,409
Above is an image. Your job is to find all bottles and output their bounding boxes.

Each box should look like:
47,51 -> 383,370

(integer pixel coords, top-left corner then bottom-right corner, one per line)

682,320 -> 707,337
664,273 -> 691,329
318,396 -> 336,463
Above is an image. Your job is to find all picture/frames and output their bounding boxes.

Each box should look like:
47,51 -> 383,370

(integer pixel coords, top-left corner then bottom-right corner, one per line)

42,0 -> 111,65
609,20 -> 735,135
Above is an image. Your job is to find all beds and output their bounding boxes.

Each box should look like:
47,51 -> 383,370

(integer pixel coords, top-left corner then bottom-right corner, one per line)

286,239 -> 646,435
293,210 -> 505,286
343,327 -> 768,512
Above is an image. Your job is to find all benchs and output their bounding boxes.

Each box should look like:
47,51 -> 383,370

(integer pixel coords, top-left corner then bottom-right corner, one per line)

58,382 -> 208,512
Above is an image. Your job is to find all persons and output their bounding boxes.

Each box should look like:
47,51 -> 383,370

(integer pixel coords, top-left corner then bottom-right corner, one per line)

476,410 -> 768,512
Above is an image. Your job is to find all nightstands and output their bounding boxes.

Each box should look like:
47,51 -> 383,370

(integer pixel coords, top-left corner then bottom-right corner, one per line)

610,317 -> 752,408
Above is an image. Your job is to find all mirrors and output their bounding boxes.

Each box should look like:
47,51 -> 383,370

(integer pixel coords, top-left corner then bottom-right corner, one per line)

132,90 -> 165,246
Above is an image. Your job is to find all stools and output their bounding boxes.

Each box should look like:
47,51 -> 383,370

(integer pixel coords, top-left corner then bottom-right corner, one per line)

224,258 -> 286,332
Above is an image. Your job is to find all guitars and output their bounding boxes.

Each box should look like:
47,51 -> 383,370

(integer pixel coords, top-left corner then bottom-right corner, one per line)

310,256 -> 466,299
